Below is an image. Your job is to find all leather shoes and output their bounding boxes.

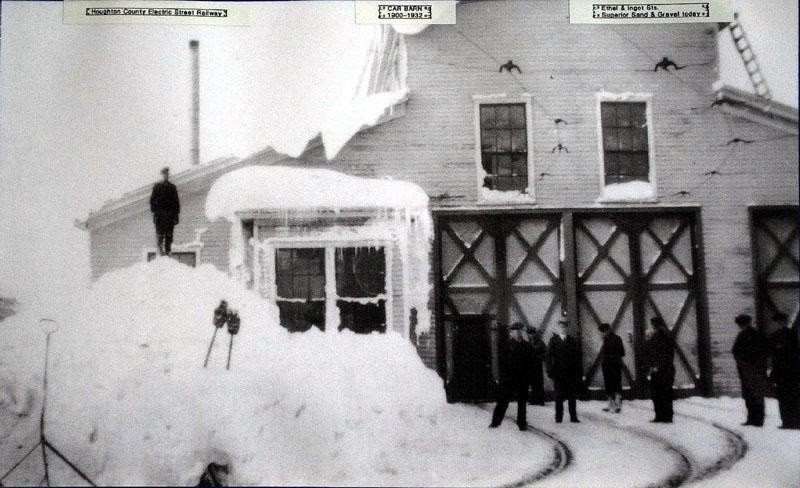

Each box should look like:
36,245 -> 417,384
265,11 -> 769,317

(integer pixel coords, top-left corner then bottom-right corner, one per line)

516,418 -> 529,432
602,407 -> 621,414
649,412 -> 674,425
555,415 -> 580,424
488,419 -> 504,430
740,416 -> 765,426
777,415 -> 799,430
158,250 -> 172,257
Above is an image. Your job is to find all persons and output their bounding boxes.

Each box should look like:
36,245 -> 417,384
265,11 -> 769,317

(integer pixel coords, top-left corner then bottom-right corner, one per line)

545,321 -> 583,422
149,167 -> 180,256
643,317 -> 675,422
488,322 -> 547,430
598,324 -> 625,413
732,312 -> 800,428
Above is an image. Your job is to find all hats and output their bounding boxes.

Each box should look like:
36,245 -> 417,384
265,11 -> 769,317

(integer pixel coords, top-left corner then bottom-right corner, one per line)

734,312 -> 791,329
161,168 -> 169,173
507,313 -> 666,336
